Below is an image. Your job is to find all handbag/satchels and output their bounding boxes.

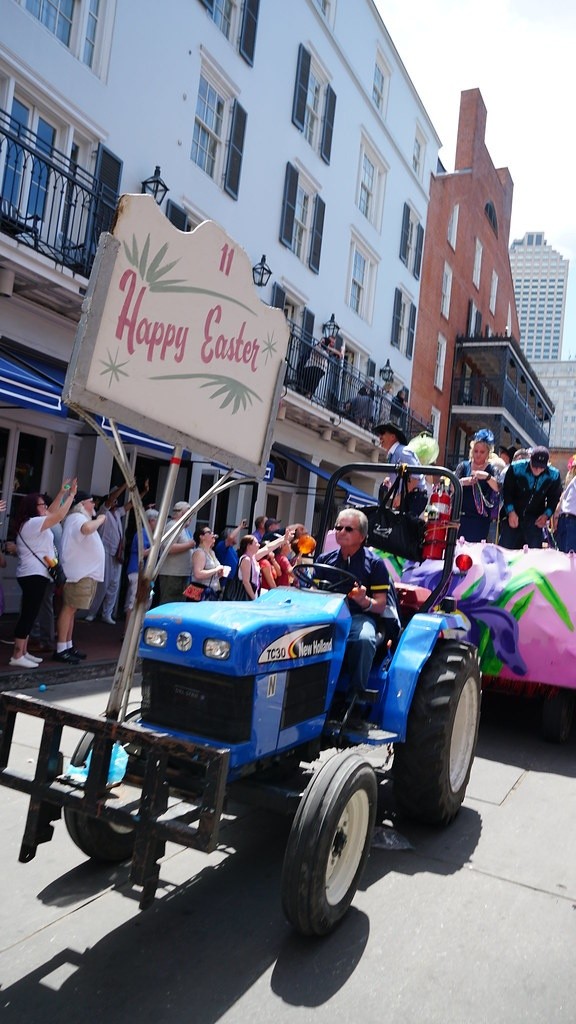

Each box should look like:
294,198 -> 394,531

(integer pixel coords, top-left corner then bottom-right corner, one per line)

366,477 -> 426,563
117,542 -> 125,564
47,564 -> 67,584
183,583 -> 210,602
222,557 -> 257,602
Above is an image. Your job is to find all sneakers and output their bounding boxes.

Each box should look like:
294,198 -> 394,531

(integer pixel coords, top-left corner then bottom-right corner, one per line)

68,647 -> 87,659
54,648 -> 79,664
8,657 -> 39,668
24,653 -> 43,663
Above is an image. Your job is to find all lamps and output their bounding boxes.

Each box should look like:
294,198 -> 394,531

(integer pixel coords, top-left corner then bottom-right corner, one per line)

322,429 -> 332,441
252,254 -> 272,287
0,268 -> 16,297
347,439 -> 356,453
140,166 -> 169,205
276,406 -> 287,421
379,358 -> 394,383
370,450 -> 379,463
322,313 -> 340,339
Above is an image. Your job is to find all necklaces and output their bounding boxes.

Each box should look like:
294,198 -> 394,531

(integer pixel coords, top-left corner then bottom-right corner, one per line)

473,464 -> 484,467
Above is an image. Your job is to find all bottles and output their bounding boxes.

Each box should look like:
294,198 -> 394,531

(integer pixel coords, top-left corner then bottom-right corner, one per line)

43,555 -> 55,568
64,481 -> 72,489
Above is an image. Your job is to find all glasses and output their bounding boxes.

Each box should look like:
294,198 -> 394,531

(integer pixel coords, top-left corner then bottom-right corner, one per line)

335,524 -> 361,532
37,503 -> 47,508
201,531 -> 212,536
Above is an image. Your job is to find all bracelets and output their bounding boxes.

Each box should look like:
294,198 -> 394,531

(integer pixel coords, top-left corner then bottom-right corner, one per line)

363,599 -> 372,612
70,492 -> 73,494
486,473 -> 492,481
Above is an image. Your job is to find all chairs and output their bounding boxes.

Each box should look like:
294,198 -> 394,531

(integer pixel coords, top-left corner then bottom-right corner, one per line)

0,195 -> 41,251
324,388 -> 346,418
284,357 -> 297,391
56,233 -> 84,274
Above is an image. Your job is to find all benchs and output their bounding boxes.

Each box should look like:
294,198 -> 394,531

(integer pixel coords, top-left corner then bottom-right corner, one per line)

329,619 -> 412,724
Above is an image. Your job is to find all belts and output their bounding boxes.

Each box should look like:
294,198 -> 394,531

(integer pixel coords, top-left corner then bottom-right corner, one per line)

561,513 -> 576,520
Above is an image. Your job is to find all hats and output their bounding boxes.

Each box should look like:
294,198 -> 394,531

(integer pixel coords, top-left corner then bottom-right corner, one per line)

372,422 -> 407,446
265,519 -> 281,529
532,446 -> 549,469
70,489 -> 93,509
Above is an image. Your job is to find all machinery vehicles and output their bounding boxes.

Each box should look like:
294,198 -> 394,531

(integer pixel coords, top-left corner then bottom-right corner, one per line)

63,462 -> 496,940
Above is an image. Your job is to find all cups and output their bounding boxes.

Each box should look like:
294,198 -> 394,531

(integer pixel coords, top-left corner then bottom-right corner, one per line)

223,566 -> 231,577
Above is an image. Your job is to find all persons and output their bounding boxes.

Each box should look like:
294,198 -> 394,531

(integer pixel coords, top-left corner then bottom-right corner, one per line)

357,376 -> 383,399
299,508 -> 399,738
373,421 -> 432,510
0,476 -> 316,670
387,389 -> 407,424
437,428 -> 576,554
298,336 -> 342,400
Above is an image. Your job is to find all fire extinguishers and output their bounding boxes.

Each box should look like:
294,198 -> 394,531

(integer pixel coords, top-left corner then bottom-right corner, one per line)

422,479 -> 450,559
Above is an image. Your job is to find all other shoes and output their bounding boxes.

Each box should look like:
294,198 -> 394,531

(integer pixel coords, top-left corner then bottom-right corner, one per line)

28,642 -> 54,653
103,616 -> 116,625
